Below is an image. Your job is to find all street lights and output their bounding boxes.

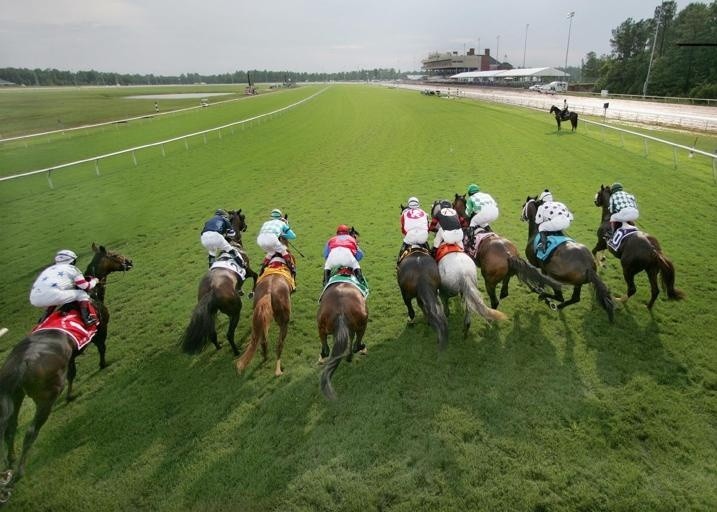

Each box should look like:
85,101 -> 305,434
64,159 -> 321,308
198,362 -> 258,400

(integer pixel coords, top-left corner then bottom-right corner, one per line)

564,11 -> 575,81
642,3 -> 671,98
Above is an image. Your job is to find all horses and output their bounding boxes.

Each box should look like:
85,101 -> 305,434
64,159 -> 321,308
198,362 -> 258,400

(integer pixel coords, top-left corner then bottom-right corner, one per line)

177,208 -> 247,356
0,241 -> 133,505
452,193 -> 568,308
317,226 -> 368,400
592,184 -> 686,308
397,204 -> 449,351
520,195 -> 615,323
431,200 -> 510,329
550,105 -> 578,132
232,213 -> 292,376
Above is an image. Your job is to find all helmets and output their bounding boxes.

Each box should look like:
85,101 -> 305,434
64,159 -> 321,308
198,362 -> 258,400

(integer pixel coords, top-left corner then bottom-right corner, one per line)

215,209 -> 228,217
440,200 -> 451,208
611,183 -> 622,192
272,209 -> 281,219
408,197 -> 419,208
54,250 -> 77,264
467,184 -> 479,195
539,191 -> 552,201
337,225 -> 347,235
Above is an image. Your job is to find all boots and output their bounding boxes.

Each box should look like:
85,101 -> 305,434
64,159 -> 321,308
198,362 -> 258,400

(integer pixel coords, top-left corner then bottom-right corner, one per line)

37,308 -> 53,322
283,254 -> 296,274
77,302 -> 97,326
209,255 -> 214,268
537,231 -> 547,250
259,257 -> 270,277
323,269 -> 330,286
354,268 -> 367,288
605,222 -> 616,239
230,249 -> 246,268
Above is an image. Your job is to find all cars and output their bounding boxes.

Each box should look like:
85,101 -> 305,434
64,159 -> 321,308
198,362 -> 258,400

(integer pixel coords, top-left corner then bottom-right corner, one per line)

543,89 -> 555,96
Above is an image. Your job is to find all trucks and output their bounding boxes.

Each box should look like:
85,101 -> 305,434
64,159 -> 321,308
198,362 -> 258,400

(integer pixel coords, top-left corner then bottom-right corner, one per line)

540,81 -> 568,94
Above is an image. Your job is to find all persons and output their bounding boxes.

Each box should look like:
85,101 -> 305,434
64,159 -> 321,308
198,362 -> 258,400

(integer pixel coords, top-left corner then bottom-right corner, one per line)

607,183 -> 640,235
323,225 -> 367,289
397,197 -> 431,265
429,200 -> 464,256
535,189 -> 574,250
561,100 -> 568,119
465,184 -> 499,246
29,249 -> 100,328
201,208 -> 247,269
257,209 -> 297,277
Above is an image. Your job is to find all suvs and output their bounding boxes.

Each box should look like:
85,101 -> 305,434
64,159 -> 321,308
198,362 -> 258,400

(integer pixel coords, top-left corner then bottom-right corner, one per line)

528,84 -> 541,92
